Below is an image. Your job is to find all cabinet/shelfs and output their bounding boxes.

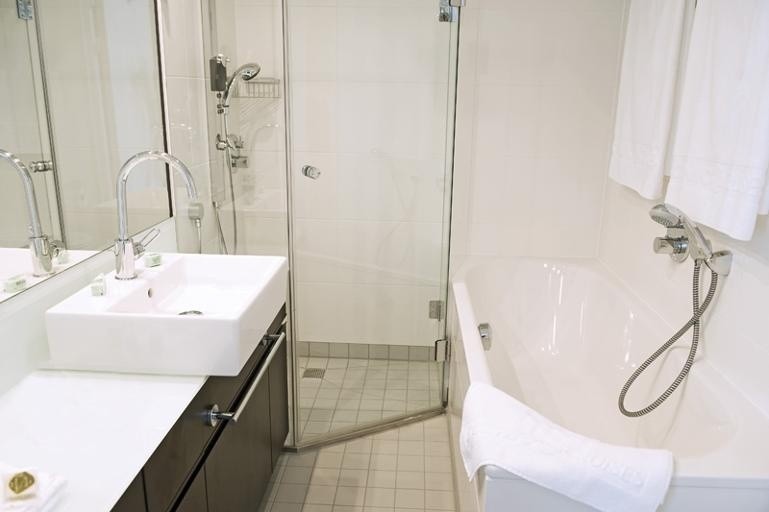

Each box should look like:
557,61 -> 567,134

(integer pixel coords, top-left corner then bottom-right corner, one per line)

5,303 -> 289,510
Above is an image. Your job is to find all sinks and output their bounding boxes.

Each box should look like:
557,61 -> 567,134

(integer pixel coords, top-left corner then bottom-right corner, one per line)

44,250 -> 291,378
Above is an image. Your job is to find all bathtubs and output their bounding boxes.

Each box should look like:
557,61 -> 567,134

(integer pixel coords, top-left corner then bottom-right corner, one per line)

433,256 -> 769,512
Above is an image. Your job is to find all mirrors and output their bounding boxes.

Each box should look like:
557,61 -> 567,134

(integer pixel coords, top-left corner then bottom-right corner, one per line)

4,3 -> 175,307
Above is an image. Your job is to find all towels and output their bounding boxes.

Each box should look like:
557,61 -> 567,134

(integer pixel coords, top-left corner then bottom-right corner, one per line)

663,2 -> 769,242
607,1 -> 696,202
459,379 -> 673,512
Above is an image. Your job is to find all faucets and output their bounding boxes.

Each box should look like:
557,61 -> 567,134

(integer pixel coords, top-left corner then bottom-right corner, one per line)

0,151 -> 63,277
114,151 -> 202,282
